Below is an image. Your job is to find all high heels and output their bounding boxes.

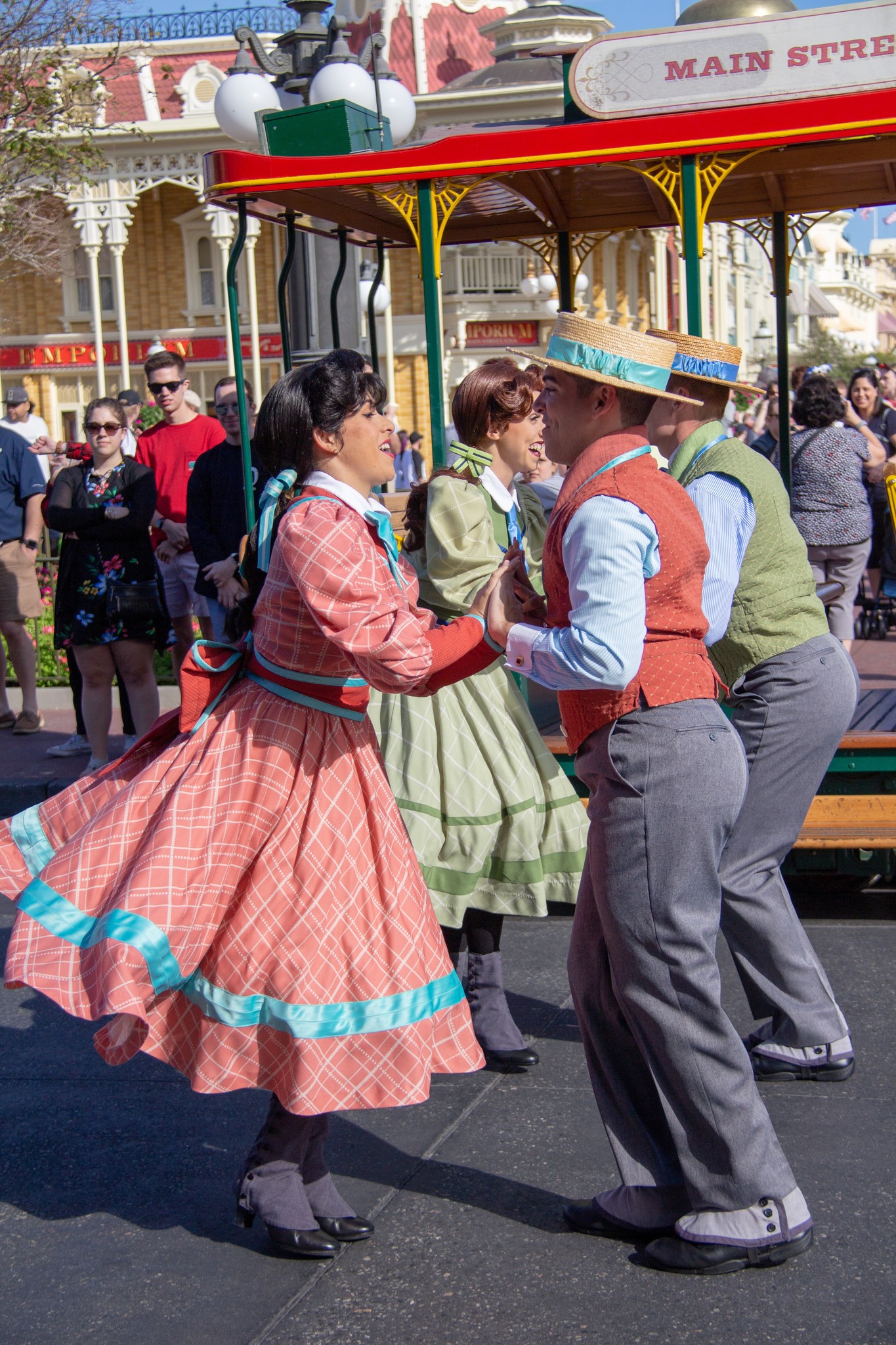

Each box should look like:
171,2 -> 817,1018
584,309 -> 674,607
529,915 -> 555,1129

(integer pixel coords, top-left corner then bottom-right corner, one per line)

313,1214 -> 375,1240
233,1194 -> 341,1255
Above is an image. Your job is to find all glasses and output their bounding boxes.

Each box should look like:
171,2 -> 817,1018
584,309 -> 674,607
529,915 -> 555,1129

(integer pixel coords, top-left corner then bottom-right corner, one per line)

853,368 -> 874,375
85,423 -> 122,435
216,401 -> 240,415
147,379 -> 185,394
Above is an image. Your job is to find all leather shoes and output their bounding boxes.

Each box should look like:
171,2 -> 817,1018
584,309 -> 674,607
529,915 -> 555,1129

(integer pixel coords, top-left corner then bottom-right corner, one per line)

482,1046 -> 539,1066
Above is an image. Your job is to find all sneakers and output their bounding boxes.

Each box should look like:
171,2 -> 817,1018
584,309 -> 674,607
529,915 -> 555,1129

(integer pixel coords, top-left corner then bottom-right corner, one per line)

47,734 -> 92,755
14,710 -> 46,732
749,1050 -> 857,1083
742,1038 -> 764,1052
563,1197 -> 671,1239
123,736 -> 138,754
642,1227 -> 813,1275
0,710 -> 15,727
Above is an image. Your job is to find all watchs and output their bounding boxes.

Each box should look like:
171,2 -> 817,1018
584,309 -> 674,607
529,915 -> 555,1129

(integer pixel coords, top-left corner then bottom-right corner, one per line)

18,537 -> 39,550
230,552 -> 241,567
154,517 -> 166,530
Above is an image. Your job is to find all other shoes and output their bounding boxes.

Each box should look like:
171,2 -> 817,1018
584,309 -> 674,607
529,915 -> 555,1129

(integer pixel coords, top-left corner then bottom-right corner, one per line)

80,756 -> 110,778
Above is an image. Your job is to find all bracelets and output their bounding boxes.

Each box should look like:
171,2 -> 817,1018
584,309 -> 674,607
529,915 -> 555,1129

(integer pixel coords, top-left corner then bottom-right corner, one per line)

105,506 -> 116,519
55,440 -> 65,455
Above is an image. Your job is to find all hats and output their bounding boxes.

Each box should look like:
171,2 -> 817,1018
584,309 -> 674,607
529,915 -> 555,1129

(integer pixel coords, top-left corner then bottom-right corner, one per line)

1,387 -> 27,403
507,313 -> 703,406
184,390 -> 202,408
117,390 -> 145,406
649,328 -> 766,395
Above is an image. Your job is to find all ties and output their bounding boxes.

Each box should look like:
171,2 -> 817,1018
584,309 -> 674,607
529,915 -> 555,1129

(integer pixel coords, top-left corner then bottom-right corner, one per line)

507,505 -> 530,572
365,511 -> 410,591
770,390 -> 779,396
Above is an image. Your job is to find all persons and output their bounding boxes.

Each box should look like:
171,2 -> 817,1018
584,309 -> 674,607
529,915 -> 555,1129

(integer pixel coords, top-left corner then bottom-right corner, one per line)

487,311 -> 817,1274
45,398 -> 170,779
639,324 -> 862,1080
718,363 -> 896,653
184,390 -> 201,414
368,359 -> 592,1070
409,431 -> 426,485
116,389 -> 146,458
0,386 -> 60,573
0,346 -> 521,1255
185,377 -> 282,643
134,351 -> 226,695
0,427 -> 46,733
391,430 -> 419,492
514,445 -> 568,525
